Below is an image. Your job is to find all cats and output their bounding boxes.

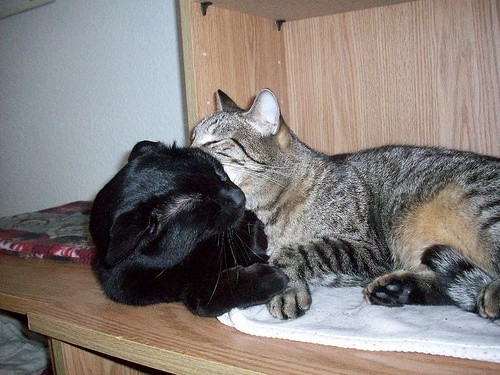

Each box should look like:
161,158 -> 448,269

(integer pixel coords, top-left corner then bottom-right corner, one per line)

87,140 -> 290,318
188,88 -> 500,322
